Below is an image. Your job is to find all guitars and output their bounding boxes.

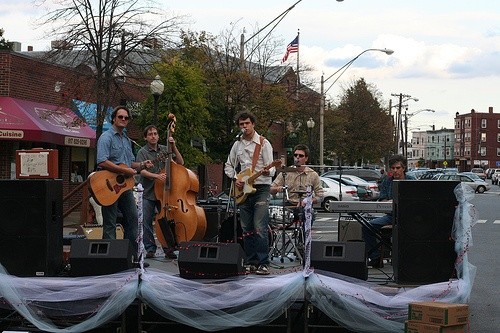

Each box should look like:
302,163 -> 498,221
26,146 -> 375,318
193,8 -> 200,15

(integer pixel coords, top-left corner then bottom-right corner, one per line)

86,150 -> 173,207
232,158 -> 282,204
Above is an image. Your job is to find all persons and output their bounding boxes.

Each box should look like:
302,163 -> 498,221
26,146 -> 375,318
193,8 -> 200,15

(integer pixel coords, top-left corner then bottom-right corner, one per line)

376,169 -> 387,191
225,113 -> 276,274
363,157 -> 418,267
137,125 -> 184,258
270,145 -> 323,208
97,106 -> 152,266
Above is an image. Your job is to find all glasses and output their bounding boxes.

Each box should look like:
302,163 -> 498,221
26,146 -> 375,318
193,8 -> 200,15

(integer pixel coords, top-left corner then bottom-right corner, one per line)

293,153 -> 306,158
116,115 -> 129,120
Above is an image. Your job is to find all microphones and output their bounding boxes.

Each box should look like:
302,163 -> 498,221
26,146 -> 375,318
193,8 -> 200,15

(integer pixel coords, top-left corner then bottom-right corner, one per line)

236,128 -> 246,137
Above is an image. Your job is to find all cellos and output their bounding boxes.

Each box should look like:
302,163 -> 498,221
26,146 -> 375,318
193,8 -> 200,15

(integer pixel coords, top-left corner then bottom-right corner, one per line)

154,112 -> 208,250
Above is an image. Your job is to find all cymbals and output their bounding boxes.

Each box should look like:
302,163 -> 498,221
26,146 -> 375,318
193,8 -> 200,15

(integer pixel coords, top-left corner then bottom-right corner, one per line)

290,190 -> 312,194
276,165 -> 304,173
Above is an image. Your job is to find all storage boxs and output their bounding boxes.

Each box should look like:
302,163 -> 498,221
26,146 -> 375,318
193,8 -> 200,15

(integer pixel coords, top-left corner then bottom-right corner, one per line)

404,302 -> 470,333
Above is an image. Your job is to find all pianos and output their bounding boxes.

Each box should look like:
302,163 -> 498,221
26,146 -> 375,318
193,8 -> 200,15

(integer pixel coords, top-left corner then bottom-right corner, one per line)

329,200 -> 393,269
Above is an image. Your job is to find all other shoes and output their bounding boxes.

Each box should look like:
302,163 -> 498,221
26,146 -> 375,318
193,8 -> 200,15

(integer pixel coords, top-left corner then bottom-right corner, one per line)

242,265 -> 254,274
146,251 -> 155,258
256,265 -> 270,274
134,261 -> 150,268
165,252 -> 177,259
294,248 -> 304,256
368,259 -> 384,268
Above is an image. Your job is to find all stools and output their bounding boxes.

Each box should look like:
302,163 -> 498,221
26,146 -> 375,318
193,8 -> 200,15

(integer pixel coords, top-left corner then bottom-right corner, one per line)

378,224 -> 393,268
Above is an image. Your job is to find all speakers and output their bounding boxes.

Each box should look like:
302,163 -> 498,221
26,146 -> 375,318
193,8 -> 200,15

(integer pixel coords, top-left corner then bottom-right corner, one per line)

74,224 -> 124,240
70,238 -> 137,277
0,178 -> 63,277
179,241 -> 247,278
310,241 -> 368,281
392,180 -> 461,284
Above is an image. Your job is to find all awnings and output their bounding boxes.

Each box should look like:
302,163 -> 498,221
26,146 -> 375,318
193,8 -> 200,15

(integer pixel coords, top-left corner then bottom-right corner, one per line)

0,96 -> 127,147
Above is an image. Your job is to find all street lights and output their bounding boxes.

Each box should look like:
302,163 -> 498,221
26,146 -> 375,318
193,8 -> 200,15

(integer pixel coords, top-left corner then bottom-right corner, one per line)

308,117 -> 315,164
319,48 -> 394,175
389,98 -> 435,161
150,75 -> 165,126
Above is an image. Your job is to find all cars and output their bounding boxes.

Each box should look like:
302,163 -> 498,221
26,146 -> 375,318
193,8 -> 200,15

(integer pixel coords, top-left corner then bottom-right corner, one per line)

406,167 -> 500,194
193,169 -> 383,220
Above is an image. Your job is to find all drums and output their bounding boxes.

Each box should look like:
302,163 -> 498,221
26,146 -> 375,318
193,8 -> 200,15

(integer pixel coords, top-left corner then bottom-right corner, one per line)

269,206 -> 295,223
218,208 -> 274,264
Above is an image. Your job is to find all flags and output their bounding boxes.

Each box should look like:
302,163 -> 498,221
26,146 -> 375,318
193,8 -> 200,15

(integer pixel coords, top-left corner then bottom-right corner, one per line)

282,36 -> 298,62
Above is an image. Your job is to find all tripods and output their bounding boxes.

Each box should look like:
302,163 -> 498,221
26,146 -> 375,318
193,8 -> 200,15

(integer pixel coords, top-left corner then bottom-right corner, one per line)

268,172 -> 303,265
